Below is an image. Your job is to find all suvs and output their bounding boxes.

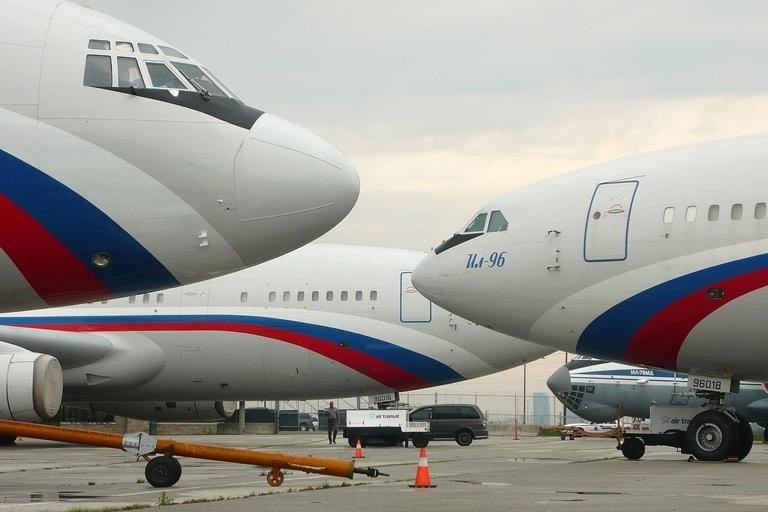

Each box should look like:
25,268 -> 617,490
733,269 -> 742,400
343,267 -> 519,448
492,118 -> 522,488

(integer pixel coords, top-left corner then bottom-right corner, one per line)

559,426 -> 586,441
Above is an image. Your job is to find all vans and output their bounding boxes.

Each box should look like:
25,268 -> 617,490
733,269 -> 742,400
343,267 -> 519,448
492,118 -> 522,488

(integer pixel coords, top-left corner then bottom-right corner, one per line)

406,400 -> 491,449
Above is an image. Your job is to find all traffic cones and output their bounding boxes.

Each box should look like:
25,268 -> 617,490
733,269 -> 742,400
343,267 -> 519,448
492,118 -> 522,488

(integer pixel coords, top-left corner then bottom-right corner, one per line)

349,439 -> 368,458
408,445 -> 437,488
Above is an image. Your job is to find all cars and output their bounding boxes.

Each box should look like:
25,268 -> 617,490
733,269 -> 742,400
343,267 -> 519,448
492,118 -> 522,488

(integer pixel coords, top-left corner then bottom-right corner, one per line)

297,412 -> 319,432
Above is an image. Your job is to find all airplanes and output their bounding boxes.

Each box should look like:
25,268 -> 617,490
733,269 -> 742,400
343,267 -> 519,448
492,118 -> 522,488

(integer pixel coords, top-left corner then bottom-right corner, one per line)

0,238 -> 564,429
402,126 -> 768,462
0,2 -> 372,429
540,349 -> 768,462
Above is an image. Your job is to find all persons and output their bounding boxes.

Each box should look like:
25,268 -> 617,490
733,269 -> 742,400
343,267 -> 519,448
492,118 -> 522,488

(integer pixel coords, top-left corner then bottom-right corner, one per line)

324,401 -> 339,445
